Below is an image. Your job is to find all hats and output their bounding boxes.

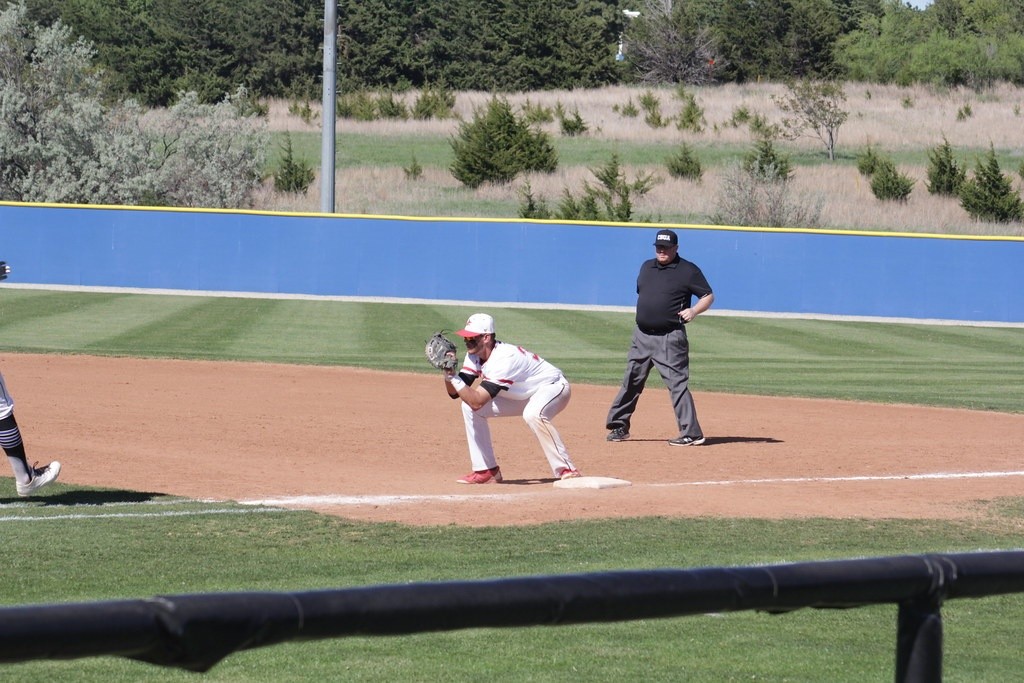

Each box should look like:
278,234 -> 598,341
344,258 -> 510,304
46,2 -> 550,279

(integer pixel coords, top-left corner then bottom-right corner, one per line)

653,230 -> 678,248
455,313 -> 495,338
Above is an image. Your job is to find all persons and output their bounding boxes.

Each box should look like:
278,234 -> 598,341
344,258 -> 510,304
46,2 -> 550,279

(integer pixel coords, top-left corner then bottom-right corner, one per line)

606,229 -> 715,447
424,313 -> 581,485
0,258 -> 61,497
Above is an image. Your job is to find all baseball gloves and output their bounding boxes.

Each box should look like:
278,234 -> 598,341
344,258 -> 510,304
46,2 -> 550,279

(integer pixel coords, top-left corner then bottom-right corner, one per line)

425,333 -> 459,371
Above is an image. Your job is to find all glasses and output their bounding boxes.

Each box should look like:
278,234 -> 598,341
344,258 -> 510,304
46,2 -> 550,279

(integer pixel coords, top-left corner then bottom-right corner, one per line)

656,244 -> 676,250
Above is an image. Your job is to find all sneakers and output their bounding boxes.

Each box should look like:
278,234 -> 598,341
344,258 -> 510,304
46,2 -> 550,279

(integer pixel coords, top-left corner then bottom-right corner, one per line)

606,427 -> 630,442
561,469 -> 581,480
669,435 -> 706,446
16,460 -> 62,496
457,466 -> 502,485
